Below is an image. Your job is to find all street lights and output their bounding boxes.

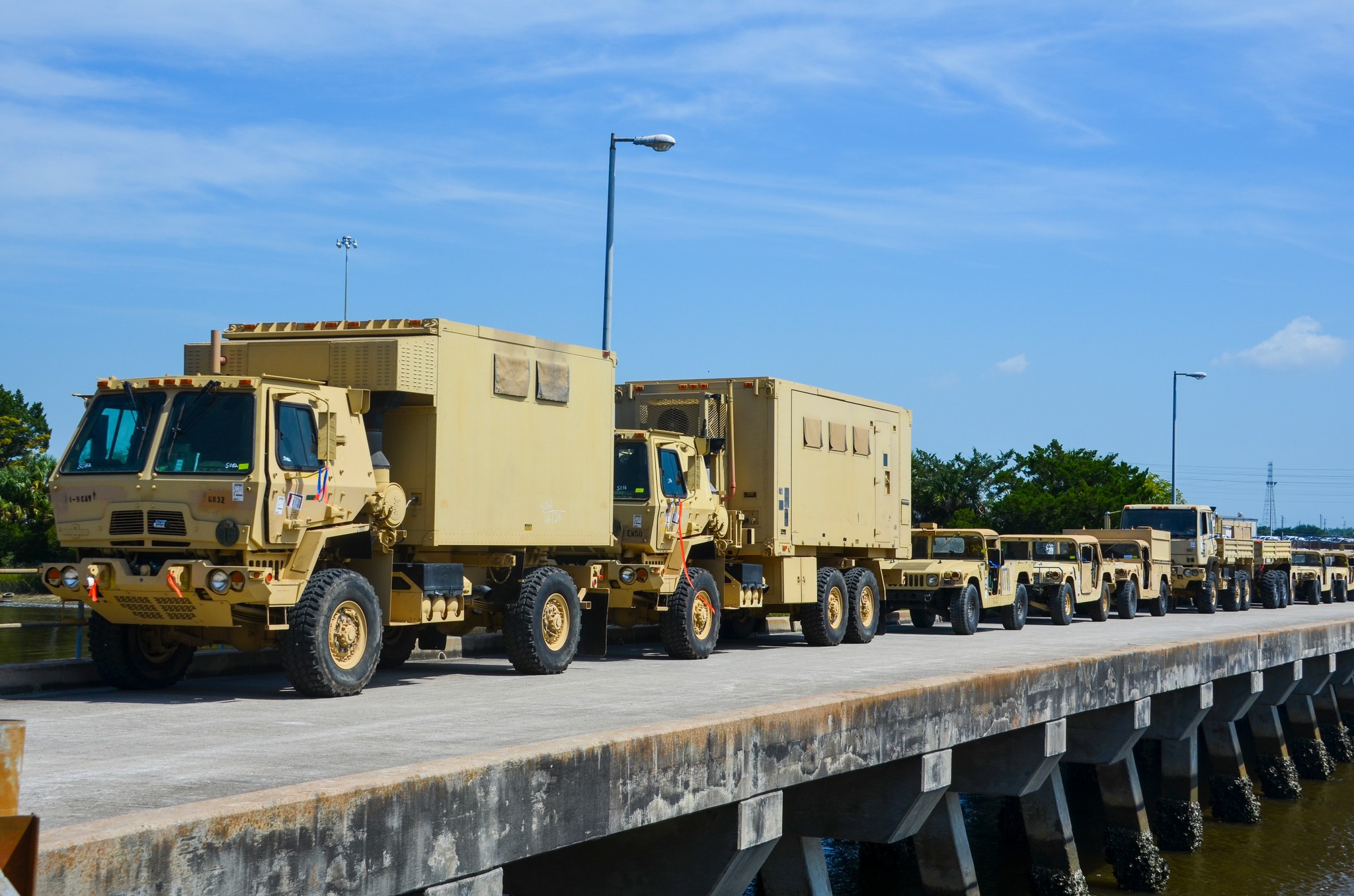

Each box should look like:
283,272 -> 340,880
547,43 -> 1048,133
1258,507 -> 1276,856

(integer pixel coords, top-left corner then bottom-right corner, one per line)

1171,371 -> 1207,505
601,131 -> 678,353
335,236 -> 358,322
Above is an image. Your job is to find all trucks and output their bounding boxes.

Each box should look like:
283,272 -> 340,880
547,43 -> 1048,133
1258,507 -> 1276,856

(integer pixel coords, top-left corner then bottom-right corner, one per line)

998,535 -> 1111,626
1062,526 -> 1172,620
491,375 -> 912,659
1104,503 -> 1293,614
884,522 -> 1035,635
39,317 -> 617,697
1290,549 -> 1354,605
